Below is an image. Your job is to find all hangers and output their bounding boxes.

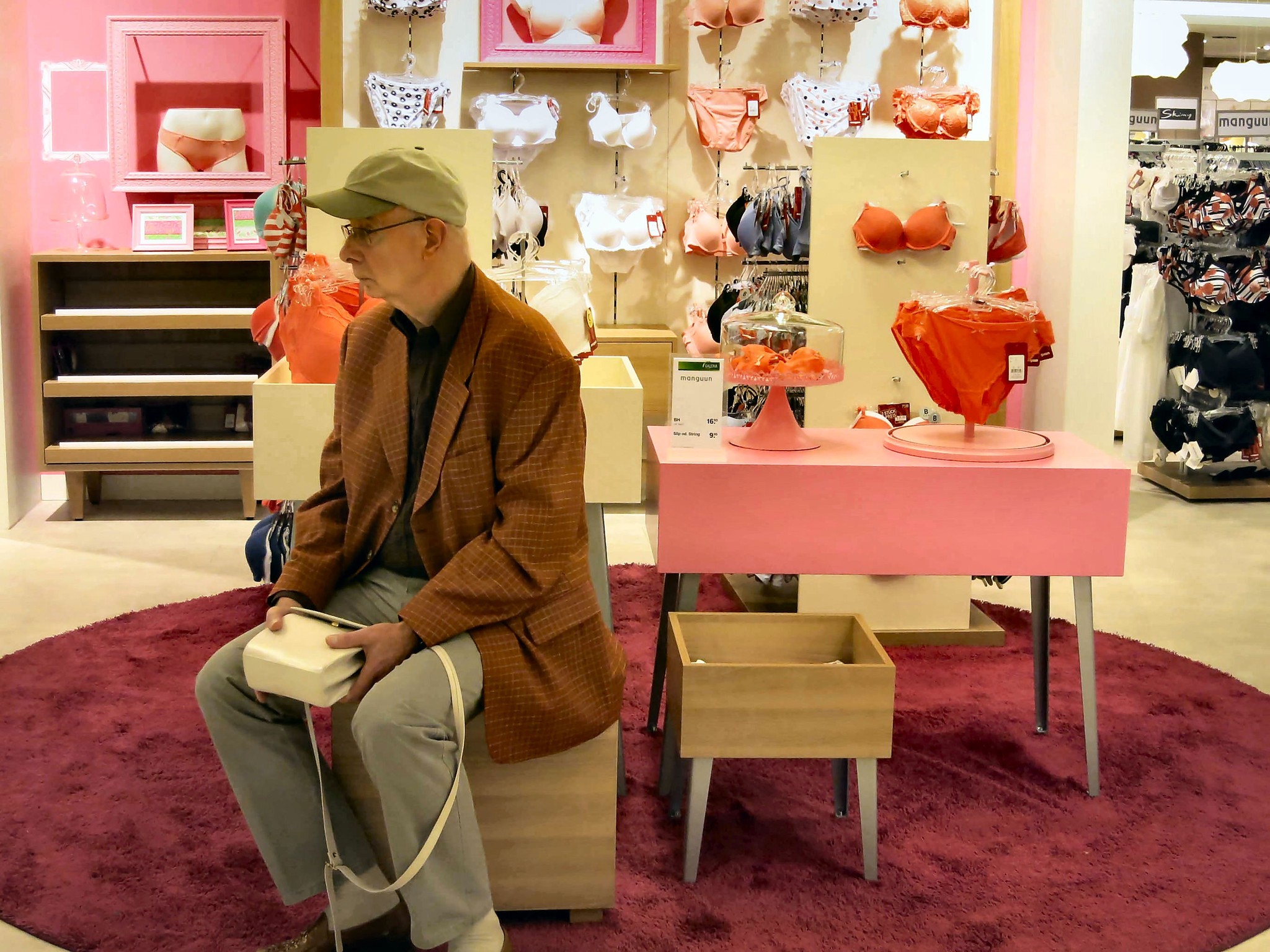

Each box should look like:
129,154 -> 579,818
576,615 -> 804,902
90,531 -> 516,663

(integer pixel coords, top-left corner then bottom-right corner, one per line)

485,230 -> 593,293
586,72 -> 653,109
685,56 -> 766,94
783,58 -> 874,96
686,174 -> 735,212
910,259 -> 1039,323
567,172 -> 662,214
366,51 -> 438,86
902,64 -> 973,98
1122,140 -> 1270,430
489,156 -> 528,206
732,258 -> 808,313
472,71 -> 561,106
746,162 -> 812,207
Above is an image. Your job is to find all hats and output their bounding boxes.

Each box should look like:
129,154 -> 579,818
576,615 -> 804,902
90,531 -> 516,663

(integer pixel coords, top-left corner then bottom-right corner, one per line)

302,146 -> 469,226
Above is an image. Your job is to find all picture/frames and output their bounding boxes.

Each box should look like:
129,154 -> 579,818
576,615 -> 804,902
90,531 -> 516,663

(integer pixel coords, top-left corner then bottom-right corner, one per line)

478,0 -> 656,64
131,203 -> 195,253
223,199 -> 270,251
106,12 -> 291,197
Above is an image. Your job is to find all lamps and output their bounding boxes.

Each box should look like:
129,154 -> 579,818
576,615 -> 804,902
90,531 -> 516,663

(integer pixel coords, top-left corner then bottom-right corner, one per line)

1210,0 -> 1270,102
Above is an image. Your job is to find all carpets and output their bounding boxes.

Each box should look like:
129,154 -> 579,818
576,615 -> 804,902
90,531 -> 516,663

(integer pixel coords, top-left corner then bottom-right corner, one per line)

0,565 -> 1270,952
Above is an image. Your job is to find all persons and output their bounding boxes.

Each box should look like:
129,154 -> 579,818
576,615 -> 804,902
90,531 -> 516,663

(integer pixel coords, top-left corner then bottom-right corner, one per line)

194,151 -> 627,952
156,108 -> 250,172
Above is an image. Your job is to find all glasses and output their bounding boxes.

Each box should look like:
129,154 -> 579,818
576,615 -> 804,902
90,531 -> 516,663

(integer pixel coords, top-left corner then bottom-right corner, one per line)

341,217 -> 426,245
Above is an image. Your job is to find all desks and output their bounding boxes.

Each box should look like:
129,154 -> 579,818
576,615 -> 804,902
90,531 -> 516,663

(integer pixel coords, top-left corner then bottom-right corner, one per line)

644,424 -> 1131,806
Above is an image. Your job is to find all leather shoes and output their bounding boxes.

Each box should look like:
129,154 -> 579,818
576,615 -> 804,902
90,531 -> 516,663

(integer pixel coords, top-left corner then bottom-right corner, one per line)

255,889 -> 410,952
500,924 -> 513,952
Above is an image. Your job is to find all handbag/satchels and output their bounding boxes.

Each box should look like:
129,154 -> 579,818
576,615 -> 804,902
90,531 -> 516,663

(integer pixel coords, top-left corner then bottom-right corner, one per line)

243,605 -> 370,709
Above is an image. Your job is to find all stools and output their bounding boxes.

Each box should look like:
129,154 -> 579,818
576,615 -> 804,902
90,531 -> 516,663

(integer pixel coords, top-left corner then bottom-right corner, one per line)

330,681 -> 618,929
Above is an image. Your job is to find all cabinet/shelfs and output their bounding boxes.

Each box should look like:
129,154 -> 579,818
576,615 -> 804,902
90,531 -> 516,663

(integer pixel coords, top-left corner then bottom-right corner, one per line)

591,321 -> 677,503
30,247 -> 292,524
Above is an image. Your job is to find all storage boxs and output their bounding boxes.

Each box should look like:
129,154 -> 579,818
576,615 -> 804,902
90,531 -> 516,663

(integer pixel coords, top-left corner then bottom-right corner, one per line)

252,349 -> 643,507
666,597 -> 897,764
